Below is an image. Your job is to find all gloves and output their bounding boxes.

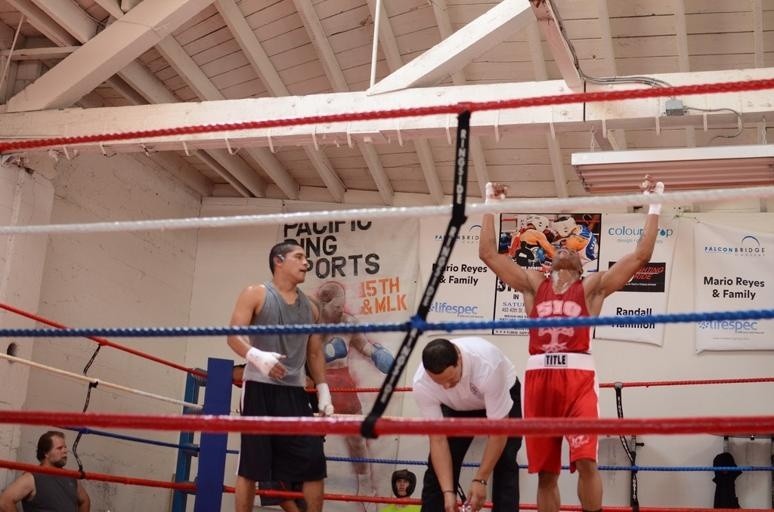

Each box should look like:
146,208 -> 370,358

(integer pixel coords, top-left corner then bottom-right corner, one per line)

371,342 -> 396,376
314,383 -> 334,417
245,346 -> 280,377
643,181 -> 666,216
320,337 -> 348,363
484,182 -> 506,215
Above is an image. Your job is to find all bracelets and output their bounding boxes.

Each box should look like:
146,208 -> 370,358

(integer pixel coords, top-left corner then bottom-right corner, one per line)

444,489 -> 455,493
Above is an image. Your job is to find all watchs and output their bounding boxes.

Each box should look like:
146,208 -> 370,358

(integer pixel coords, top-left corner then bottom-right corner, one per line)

472,479 -> 488,486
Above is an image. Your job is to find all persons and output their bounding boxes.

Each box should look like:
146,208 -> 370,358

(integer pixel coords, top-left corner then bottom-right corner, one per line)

479,175 -> 664,511
1,432 -> 91,512
379,469 -> 422,512
509,214 -> 600,272
307,280 -> 396,512
227,243 -> 334,512
412,335 -> 524,511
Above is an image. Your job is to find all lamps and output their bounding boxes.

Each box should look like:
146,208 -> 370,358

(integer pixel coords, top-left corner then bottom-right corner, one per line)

571,144 -> 774,194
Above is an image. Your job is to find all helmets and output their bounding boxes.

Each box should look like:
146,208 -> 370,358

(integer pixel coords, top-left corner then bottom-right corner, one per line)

525,214 -> 549,234
552,216 -> 577,238
391,470 -> 416,498
